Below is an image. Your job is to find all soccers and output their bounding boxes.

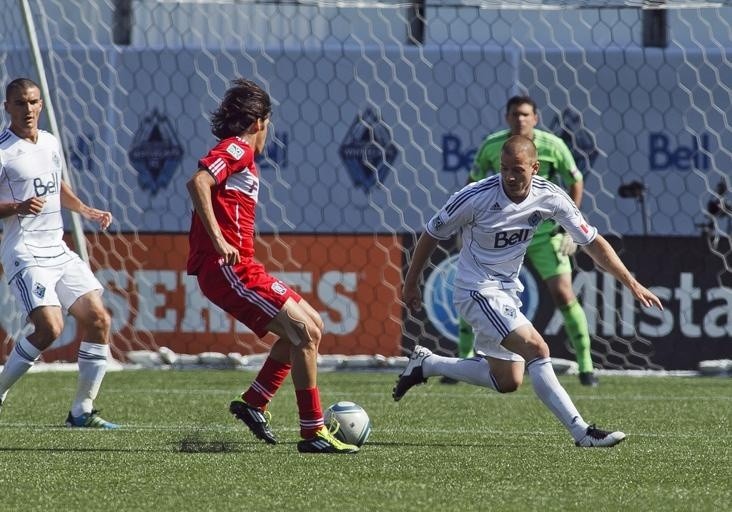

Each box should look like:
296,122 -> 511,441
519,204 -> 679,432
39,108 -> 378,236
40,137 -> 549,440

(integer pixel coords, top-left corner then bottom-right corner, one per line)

324,402 -> 370,448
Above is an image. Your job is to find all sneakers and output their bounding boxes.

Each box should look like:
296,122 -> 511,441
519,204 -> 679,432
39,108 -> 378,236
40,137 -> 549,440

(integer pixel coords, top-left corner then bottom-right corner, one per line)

65,409 -> 121,431
226,390 -> 281,446
390,342 -> 435,404
572,423 -> 626,449
578,370 -> 598,388
295,424 -> 362,456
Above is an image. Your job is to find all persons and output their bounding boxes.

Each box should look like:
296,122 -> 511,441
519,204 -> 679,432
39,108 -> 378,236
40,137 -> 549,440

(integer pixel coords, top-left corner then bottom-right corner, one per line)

0,79 -> 120,430
392,135 -> 663,446
439,94 -> 600,383
187,76 -> 360,454
